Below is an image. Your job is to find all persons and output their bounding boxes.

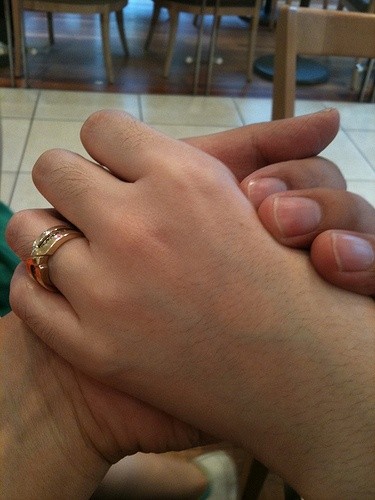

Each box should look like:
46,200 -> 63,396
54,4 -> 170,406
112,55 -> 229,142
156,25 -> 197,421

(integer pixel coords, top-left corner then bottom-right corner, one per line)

0,107 -> 375,500
6,108 -> 375,500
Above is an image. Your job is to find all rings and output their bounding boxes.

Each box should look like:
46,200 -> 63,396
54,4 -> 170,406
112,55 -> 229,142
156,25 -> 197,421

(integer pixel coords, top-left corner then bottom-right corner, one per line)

26,224 -> 84,293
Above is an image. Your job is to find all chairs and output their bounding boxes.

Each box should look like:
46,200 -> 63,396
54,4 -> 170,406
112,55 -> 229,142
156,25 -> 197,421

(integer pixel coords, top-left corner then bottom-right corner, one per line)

145,0 -> 261,85
11,0 -> 131,84
271,0 -> 375,121
